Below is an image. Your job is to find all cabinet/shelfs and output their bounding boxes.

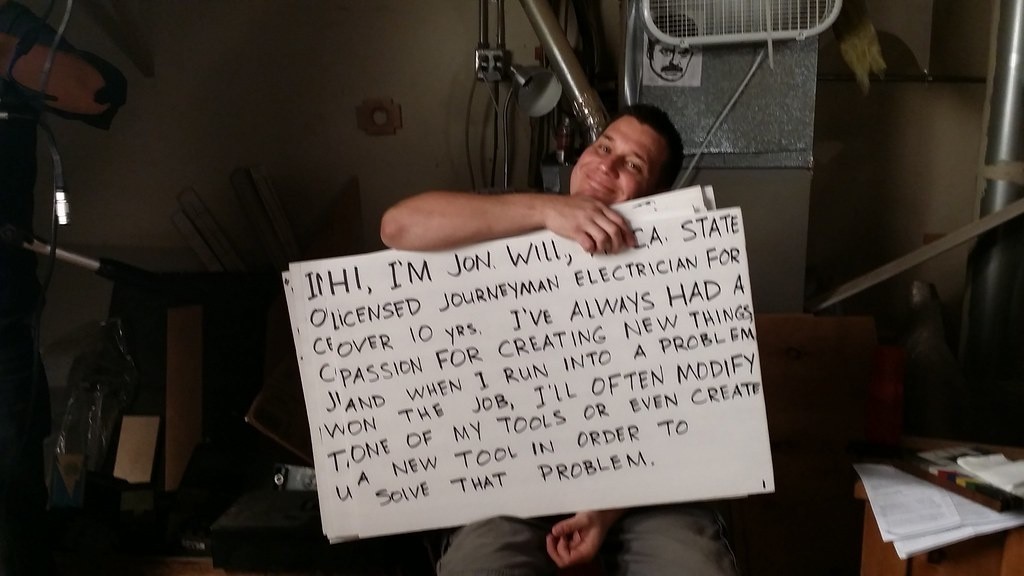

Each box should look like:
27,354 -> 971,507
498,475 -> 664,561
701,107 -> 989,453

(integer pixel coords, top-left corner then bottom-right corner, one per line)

855,480 -> 1023,576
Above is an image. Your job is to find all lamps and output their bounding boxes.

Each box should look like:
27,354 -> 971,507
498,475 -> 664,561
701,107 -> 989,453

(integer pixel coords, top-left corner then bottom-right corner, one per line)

509,62 -> 562,120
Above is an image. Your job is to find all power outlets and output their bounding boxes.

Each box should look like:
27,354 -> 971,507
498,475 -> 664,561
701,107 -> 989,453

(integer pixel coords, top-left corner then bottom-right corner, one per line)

474,50 -> 505,80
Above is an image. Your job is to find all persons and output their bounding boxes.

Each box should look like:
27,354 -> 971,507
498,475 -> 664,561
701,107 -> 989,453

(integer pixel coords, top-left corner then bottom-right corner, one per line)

0,1 -> 115,576
378,102 -> 736,576
